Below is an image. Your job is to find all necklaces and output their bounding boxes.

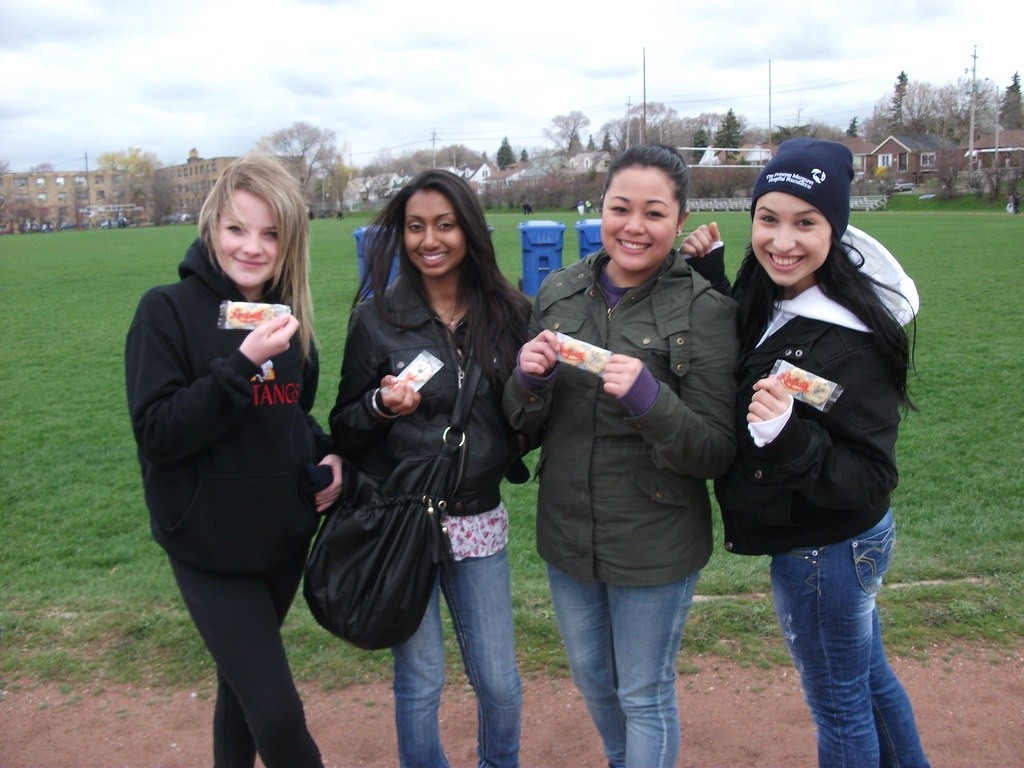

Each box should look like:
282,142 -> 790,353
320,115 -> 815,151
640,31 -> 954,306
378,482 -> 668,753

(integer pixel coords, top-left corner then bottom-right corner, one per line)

433,304 -> 464,325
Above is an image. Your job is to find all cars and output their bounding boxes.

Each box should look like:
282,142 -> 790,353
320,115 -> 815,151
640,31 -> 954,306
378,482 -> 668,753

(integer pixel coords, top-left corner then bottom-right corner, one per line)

41,224 -> 54,232
157,213 -> 192,225
24,223 -> 41,231
101,218 -> 127,228
891,179 -> 912,191
61,222 -> 76,230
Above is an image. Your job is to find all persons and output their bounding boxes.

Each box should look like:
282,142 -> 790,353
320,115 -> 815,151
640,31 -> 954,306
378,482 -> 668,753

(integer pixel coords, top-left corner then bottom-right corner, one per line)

522,201 -> 533,215
577,199 -> 593,216
1007,192 -> 1020,215
678,138 -> 933,768
327,170 -> 533,768
124,158 -> 343,768
502,143 -> 739,768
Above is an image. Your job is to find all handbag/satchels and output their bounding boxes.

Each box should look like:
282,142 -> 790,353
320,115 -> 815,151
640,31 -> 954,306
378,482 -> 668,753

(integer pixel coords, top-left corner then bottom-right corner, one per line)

301,455 -> 454,652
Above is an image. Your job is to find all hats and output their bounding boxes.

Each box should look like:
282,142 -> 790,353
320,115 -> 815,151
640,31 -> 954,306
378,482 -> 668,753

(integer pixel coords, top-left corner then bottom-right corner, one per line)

750,136 -> 855,244
